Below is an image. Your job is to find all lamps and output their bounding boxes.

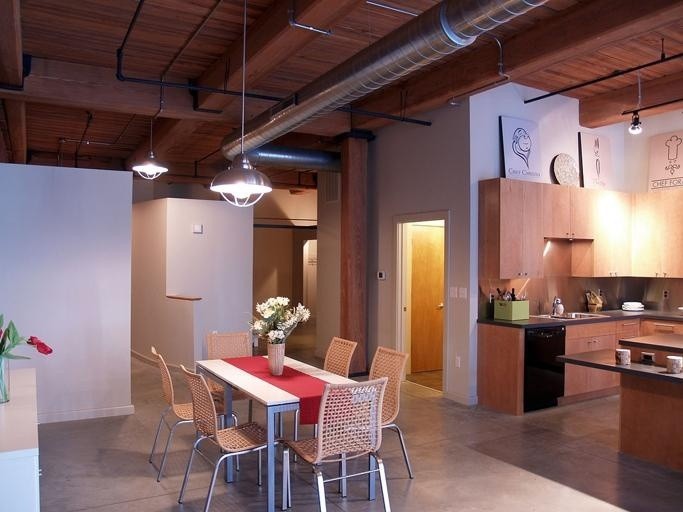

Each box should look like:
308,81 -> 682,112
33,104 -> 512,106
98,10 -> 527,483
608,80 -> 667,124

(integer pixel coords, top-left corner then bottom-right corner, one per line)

628,112 -> 643,134
132,1 -> 272,207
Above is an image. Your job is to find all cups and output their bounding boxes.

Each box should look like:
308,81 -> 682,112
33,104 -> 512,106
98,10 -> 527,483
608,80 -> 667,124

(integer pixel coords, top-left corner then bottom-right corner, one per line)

615,348 -> 631,366
666,355 -> 683,374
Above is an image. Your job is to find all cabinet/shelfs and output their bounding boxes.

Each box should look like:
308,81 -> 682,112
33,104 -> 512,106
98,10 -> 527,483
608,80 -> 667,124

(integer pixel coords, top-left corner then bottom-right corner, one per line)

631,189 -> 683,279
542,183 -> 593,241
478,177 -> 543,280
564,317 -> 683,399
571,188 -> 631,279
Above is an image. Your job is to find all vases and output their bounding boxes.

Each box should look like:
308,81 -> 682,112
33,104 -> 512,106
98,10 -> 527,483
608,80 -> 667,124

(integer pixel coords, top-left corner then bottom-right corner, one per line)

0,354 -> 10,404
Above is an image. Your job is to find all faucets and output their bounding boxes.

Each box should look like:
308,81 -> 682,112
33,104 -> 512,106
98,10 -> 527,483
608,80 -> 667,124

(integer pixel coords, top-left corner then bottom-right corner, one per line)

552,296 -> 562,315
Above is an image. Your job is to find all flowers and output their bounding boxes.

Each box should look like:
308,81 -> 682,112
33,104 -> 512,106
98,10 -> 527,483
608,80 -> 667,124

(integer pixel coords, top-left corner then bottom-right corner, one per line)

0,313 -> 53,400
247,296 -> 310,343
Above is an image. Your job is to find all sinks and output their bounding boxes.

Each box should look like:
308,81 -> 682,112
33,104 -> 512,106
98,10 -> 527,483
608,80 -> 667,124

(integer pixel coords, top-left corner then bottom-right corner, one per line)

530,312 -> 576,322
551,310 -> 612,320
